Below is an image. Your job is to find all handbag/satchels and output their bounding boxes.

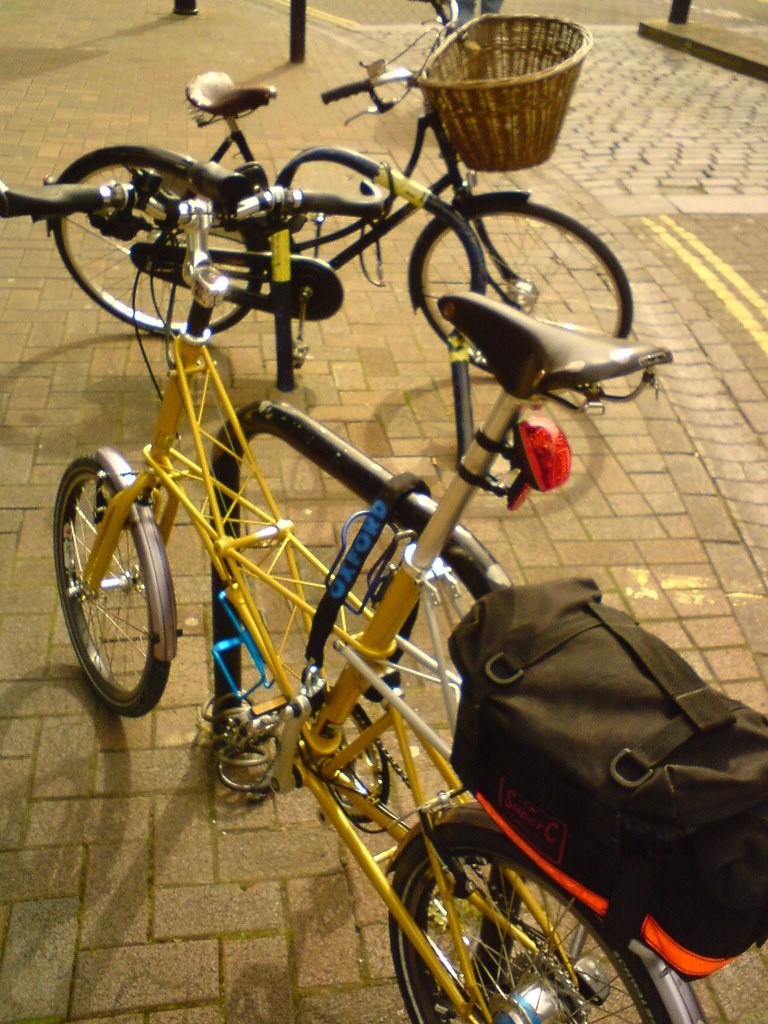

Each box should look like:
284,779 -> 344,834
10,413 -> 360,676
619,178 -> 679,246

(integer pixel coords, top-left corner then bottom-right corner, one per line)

450,570 -> 767,982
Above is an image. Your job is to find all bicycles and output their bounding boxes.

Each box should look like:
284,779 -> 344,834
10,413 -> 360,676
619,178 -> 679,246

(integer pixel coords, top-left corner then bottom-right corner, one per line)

41,0 -> 636,375
0,167 -> 712,1024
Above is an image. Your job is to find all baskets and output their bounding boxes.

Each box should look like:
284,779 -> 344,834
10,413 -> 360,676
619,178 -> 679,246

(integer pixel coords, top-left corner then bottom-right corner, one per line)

414,14 -> 593,171
